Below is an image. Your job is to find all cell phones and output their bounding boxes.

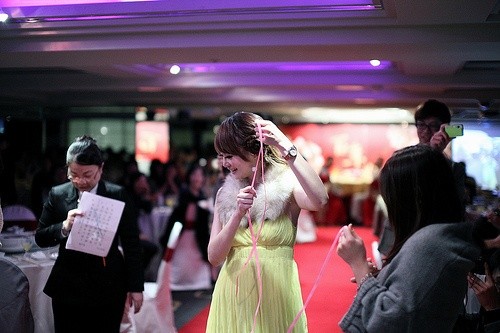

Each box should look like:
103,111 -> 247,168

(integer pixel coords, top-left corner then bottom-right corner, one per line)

445,124 -> 463,137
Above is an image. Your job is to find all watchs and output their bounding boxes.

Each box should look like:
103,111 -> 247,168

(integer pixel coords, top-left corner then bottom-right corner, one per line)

283,146 -> 298,160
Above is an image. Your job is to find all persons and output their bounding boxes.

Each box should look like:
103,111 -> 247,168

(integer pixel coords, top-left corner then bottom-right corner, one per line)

297,98 -> 500,333
206,110 -> 328,333
0,135 -> 231,333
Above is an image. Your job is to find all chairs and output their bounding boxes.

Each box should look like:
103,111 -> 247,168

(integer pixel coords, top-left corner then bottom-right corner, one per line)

128,221 -> 182,333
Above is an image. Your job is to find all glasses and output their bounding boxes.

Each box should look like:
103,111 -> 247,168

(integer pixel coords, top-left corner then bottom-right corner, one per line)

67,166 -> 101,182
415,121 -> 443,131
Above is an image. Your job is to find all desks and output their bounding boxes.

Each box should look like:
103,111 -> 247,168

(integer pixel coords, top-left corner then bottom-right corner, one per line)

0,244 -> 60,333
